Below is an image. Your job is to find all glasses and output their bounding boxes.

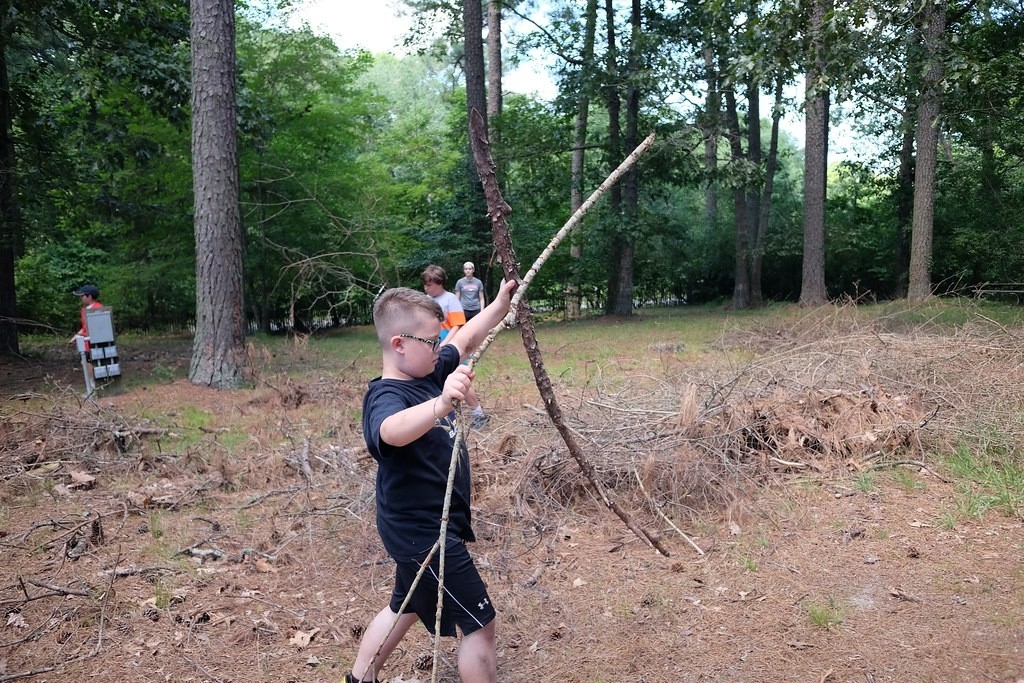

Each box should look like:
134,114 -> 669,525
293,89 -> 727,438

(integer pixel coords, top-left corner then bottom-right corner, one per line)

399,334 -> 441,352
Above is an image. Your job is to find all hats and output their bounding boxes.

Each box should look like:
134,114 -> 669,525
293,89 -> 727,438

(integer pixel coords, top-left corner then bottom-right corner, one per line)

73,285 -> 100,299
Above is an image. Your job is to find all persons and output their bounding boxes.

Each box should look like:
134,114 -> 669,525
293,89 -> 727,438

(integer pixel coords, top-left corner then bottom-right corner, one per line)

343,276 -> 517,683
66,283 -> 104,400
454,262 -> 485,325
420,265 -> 488,440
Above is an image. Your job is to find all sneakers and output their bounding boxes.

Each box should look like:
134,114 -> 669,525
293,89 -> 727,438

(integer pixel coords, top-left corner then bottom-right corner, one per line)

468,413 -> 491,430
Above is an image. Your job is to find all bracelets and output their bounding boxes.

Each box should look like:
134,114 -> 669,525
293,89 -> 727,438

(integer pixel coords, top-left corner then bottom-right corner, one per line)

433,395 -> 447,426
